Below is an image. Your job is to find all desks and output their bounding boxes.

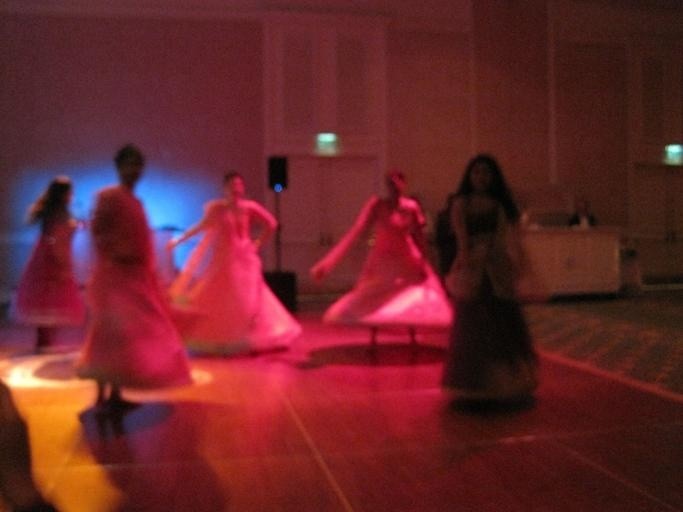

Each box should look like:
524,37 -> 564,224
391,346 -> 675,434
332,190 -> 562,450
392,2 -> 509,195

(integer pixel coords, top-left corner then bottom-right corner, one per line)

515,225 -> 621,304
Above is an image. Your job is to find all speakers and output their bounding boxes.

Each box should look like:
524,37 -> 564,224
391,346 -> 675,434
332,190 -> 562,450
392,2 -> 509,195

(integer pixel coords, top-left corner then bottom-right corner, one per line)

264,271 -> 298,313
268,156 -> 288,191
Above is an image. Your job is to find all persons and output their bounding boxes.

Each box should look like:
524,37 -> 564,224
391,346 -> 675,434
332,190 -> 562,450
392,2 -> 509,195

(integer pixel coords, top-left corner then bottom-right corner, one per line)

75,140 -> 195,415
441,153 -> 538,399
435,193 -> 596,275
310,170 -> 458,354
165,172 -> 302,354
9,176 -> 94,348
1,381 -> 58,512
